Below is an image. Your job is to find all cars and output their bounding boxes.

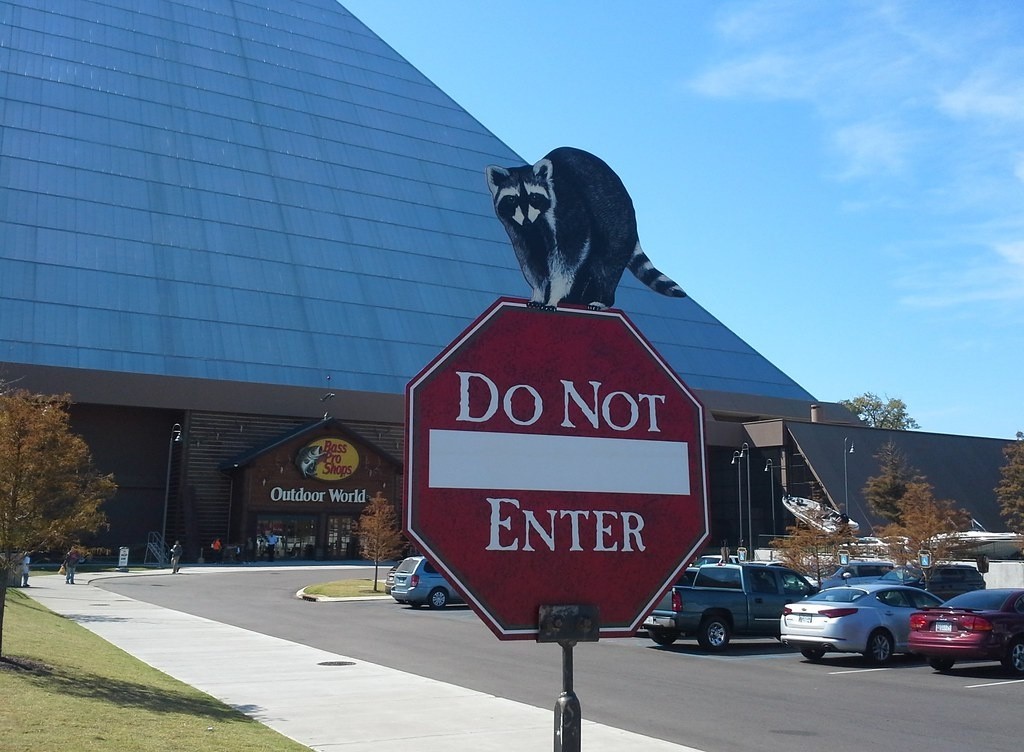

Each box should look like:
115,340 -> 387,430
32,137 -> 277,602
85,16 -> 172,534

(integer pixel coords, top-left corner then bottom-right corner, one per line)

907,588 -> 1024,678
780,585 -> 946,664
689,555 -> 741,568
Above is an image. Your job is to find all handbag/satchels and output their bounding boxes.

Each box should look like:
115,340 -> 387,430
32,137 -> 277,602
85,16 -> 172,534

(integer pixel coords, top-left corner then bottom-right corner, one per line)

211,544 -> 213,548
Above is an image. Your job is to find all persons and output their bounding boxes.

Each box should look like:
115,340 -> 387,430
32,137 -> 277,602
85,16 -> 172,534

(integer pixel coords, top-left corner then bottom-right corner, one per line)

210,537 -> 222,564
171,541 -> 182,574
23,552 -> 31,587
61,545 -> 80,584
267,532 -> 277,562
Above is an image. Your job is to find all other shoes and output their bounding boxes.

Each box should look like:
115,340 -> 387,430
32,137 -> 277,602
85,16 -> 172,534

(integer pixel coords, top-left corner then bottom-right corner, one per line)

172,571 -> 176,574
66,580 -> 69,584
23,583 -> 30,587
71,582 -> 75,584
177,567 -> 180,572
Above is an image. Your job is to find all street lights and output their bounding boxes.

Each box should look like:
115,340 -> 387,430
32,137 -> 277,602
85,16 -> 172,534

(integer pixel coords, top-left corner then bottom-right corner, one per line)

731,450 -> 743,549
763,459 -> 777,542
844,436 -> 855,516
740,443 -> 754,561
159,423 -> 184,565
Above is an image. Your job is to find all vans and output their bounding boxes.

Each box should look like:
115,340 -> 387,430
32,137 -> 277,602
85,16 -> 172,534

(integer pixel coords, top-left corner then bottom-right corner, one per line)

820,561 -> 896,589
872,564 -> 987,604
384,556 -> 468,609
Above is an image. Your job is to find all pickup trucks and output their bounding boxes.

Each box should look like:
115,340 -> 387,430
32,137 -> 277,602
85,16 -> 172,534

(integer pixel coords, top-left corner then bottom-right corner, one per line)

641,564 -> 820,650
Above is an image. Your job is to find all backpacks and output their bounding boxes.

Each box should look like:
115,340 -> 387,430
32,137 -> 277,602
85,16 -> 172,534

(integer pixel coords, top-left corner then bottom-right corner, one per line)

69,551 -> 80,564
